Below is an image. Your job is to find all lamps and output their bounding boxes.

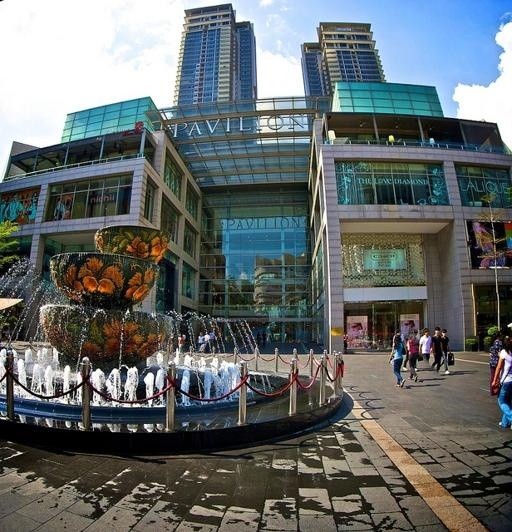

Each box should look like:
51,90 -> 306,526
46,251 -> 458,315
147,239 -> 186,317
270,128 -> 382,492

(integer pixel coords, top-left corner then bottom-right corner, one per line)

388,135 -> 395,145
429,137 -> 435,148
328,129 -> 337,144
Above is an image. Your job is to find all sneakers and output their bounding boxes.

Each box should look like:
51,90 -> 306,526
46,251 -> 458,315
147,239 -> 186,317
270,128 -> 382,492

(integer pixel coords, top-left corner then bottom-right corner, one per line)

499,421 -> 512,430
395,366 -> 451,388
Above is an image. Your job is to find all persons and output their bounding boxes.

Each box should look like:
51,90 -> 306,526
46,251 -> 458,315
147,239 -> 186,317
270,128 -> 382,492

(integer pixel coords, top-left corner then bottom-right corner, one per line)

178,330 -> 222,353
257,331 -> 266,346
489,331 -> 512,430
388,327 -> 451,388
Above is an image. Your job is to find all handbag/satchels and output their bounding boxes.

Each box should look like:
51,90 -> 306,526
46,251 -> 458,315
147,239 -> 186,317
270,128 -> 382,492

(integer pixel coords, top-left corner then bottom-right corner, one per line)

491,379 -> 501,397
448,353 -> 455,366
